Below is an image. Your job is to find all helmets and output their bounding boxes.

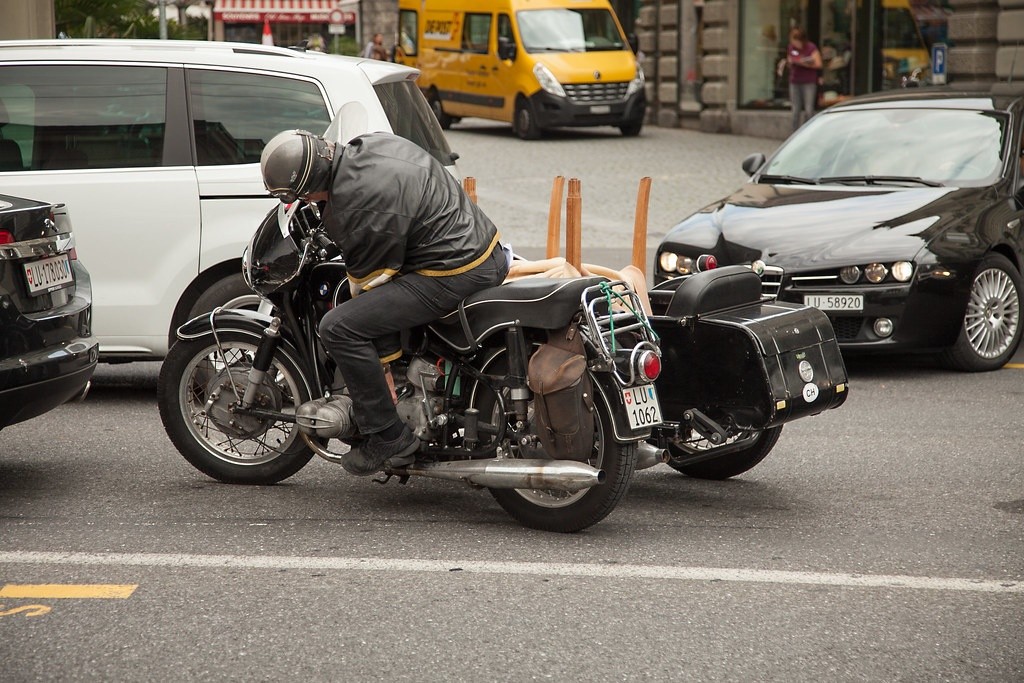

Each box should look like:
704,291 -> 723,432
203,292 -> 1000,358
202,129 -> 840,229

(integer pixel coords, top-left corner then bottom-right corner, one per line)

260,128 -> 330,205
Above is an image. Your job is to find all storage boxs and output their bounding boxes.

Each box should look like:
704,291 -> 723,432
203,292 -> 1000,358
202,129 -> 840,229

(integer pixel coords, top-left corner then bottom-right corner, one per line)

701,302 -> 849,428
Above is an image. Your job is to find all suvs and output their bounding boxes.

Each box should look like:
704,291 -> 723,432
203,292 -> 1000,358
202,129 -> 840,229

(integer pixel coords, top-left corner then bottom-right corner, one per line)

0,39 -> 460,368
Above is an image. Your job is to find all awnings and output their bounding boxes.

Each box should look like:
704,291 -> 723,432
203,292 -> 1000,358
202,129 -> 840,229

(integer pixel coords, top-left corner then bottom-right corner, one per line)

212,0 -> 354,24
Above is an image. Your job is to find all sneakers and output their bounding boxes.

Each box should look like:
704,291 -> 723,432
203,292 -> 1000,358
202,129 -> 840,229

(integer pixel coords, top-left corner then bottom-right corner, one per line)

341,426 -> 422,475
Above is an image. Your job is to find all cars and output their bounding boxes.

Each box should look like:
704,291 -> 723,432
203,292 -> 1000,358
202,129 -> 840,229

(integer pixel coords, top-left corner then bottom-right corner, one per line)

651,82 -> 1023,374
0,195 -> 100,433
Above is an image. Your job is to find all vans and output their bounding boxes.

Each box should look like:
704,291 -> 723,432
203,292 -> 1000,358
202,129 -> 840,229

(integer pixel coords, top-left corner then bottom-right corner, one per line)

391,0 -> 649,140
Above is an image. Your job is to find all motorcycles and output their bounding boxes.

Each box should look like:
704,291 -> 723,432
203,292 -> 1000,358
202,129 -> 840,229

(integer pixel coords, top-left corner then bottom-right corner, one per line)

156,103 -> 852,535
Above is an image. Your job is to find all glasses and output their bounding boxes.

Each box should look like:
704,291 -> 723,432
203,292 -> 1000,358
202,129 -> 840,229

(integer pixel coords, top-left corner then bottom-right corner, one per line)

297,193 -> 312,202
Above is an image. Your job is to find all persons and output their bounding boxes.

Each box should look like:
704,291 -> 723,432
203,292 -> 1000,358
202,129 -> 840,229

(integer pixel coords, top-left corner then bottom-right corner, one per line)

776,27 -> 850,134
298,24 -> 417,63
260,131 -> 507,476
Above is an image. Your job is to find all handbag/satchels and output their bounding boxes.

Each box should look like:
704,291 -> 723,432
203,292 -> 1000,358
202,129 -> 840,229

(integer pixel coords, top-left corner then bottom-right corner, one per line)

526,323 -> 595,460
